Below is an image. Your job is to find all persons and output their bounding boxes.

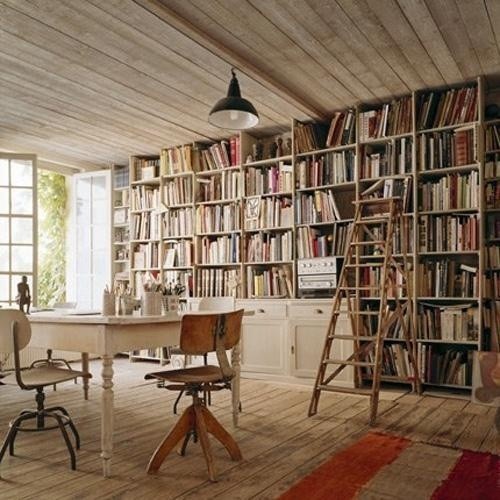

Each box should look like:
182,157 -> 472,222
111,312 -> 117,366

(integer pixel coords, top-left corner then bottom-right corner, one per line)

18,276 -> 31,315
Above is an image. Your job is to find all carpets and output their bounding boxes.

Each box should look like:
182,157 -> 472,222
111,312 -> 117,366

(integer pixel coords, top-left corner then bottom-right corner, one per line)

274,431 -> 500,499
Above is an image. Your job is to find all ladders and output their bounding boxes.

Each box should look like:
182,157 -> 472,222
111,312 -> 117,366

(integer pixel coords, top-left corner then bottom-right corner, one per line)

308,197 -> 423,427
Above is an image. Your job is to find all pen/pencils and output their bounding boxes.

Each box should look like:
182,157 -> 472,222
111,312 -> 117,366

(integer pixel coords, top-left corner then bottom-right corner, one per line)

103,281 -> 133,296
143,277 -> 185,295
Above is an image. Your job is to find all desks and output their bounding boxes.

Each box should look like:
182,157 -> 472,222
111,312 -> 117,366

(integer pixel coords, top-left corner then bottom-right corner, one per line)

18,305 -> 188,462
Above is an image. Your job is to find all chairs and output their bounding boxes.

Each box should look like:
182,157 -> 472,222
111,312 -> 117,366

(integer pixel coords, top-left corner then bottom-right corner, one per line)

30,349 -> 78,392
139,309 -> 245,477
1,306 -> 93,473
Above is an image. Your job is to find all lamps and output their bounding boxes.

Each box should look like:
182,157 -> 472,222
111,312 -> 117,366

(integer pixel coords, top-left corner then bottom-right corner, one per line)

207,65 -> 259,130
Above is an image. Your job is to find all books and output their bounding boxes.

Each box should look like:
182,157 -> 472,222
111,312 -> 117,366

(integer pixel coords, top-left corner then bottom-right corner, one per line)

293,109 -> 357,300
356,84 -> 499,386
241,160 -> 294,300
133,133 -> 241,298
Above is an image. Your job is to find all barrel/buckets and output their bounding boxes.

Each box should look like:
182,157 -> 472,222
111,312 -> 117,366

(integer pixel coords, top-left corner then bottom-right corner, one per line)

141,292 -> 163,314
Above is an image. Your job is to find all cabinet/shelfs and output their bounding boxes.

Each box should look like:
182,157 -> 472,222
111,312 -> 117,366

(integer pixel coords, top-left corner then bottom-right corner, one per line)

111,86 -> 499,392
